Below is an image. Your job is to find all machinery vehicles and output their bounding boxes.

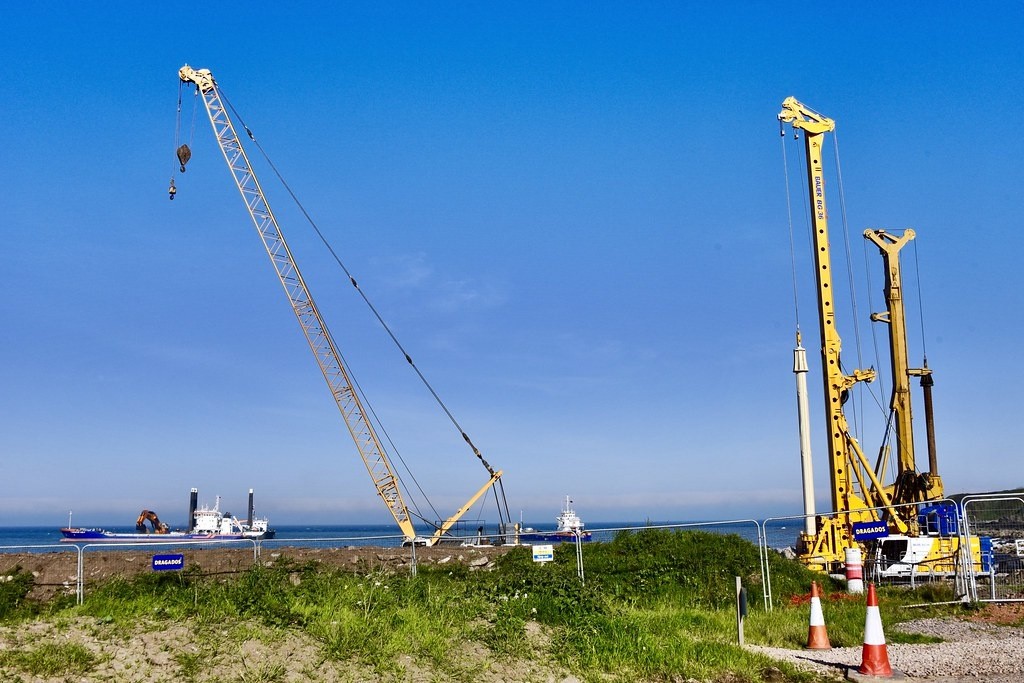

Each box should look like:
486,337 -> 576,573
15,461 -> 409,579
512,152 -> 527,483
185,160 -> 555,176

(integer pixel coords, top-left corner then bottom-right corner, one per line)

776,95 -> 992,583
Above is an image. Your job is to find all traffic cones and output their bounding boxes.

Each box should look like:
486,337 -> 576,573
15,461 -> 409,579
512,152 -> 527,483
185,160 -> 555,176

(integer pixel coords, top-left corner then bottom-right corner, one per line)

803,579 -> 835,652
847,582 -> 911,683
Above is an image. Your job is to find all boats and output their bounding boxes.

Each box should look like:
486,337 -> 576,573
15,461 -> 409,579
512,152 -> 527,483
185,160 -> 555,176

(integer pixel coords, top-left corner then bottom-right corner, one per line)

60,487 -> 278,545
512,493 -> 592,543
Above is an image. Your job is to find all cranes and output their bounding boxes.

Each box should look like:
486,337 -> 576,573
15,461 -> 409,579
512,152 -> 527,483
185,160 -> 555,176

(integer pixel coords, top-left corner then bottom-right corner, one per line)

165,66 -> 521,549
